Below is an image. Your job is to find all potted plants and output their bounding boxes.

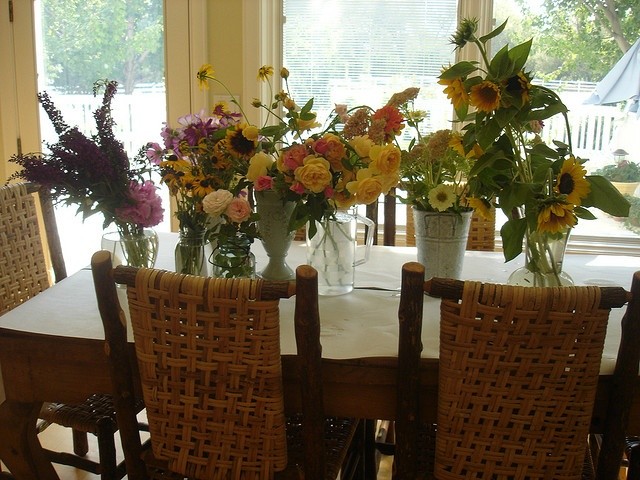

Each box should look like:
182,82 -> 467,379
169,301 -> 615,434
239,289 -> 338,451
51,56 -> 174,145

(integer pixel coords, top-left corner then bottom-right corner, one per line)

602,160 -> 640,196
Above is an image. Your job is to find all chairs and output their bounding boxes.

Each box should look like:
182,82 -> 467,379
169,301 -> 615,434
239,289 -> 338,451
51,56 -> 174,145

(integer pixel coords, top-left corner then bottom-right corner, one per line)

384,179 -> 494,250
389,262 -> 640,478
247,179 -> 378,243
90,250 -> 377,479
1,183 -> 151,480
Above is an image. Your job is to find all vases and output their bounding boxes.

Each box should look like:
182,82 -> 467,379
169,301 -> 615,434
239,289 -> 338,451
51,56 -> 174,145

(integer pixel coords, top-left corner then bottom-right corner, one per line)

503,224 -> 576,290
212,237 -> 255,281
410,205 -> 474,284
97,231 -> 158,290
173,230 -> 209,278
253,186 -> 301,285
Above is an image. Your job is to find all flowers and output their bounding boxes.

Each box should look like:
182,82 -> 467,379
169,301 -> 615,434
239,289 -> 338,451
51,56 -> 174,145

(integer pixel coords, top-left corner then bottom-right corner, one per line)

438,15 -> 633,285
133,109 -> 226,268
196,120 -> 260,241
3,78 -> 165,267
247,103 -> 404,237
196,64 -> 318,192
389,87 -> 486,213
